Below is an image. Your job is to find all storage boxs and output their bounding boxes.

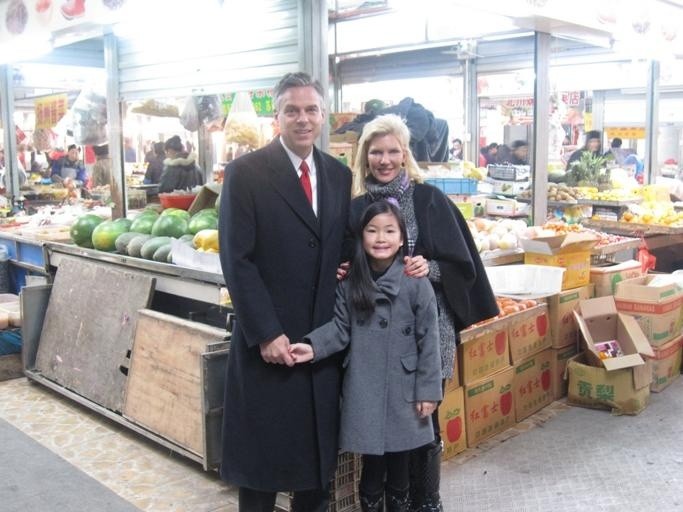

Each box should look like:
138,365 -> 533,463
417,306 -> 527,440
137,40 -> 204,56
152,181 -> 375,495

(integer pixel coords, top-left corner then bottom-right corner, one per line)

435,396 -> 466,456
516,234 -> 590,282
629,286 -> 683,339
499,297 -> 552,353
512,361 -> 559,414
648,340 -> 683,391
559,361 -> 652,410
574,304 -> 642,362
451,311 -> 506,376
591,259 -> 643,293
555,291 -> 598,349
461,382 -> 518,435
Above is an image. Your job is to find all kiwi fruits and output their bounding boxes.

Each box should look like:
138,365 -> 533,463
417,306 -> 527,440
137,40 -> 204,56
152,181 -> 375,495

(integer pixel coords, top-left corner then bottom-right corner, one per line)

520,182 -> 580,202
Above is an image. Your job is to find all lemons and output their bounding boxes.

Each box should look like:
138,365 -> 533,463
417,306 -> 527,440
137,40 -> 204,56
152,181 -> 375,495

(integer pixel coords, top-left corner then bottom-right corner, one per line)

621,205 -> 683,228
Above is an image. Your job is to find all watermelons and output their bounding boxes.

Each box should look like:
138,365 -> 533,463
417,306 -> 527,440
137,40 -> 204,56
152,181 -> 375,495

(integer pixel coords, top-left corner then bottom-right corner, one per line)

66,203 -> 220,266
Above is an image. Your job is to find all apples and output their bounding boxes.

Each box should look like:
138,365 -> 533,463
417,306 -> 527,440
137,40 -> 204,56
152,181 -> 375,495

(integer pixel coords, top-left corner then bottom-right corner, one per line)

493,331 -> 506,354
446,416 -> 462,442
465,214 -> 525,254
536,313 -> 547,337
542,369 -> 551,390
499,391 -> 512,414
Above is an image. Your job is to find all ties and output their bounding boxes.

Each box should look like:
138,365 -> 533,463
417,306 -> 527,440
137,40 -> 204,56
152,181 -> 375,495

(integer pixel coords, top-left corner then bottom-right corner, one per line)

300,161 -> 312,205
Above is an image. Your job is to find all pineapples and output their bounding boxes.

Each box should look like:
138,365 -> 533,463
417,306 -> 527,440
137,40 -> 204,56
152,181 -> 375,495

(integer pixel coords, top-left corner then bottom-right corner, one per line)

569,146 -> 613,191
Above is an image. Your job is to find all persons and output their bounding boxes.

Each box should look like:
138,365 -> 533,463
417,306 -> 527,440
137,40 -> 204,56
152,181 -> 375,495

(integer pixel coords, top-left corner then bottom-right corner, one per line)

602,138 -> 625,161
124,134 -> 203,195
218,71 -> 353,511
288,199 -> 443,512
0,143 -> 112,191
335,113 -> 499,511
565,130 -> 604,171
449,139 -> 530,169
